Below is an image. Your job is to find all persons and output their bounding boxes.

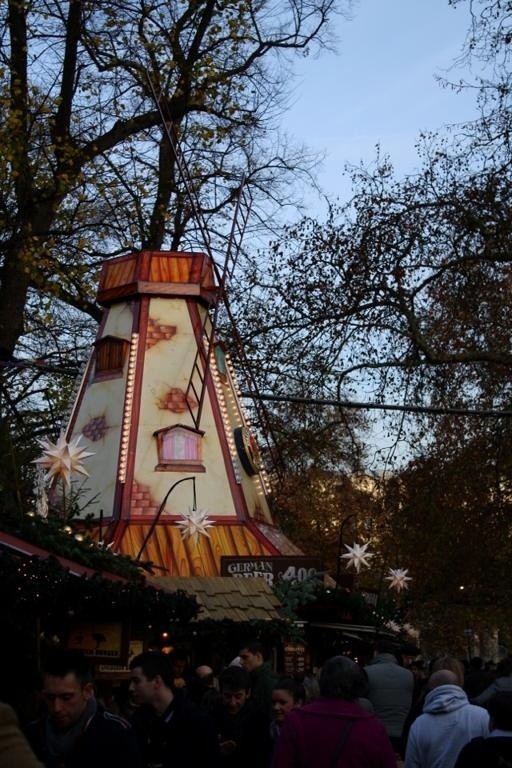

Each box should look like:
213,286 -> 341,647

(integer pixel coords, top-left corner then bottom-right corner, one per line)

0,644 -> 512,768
